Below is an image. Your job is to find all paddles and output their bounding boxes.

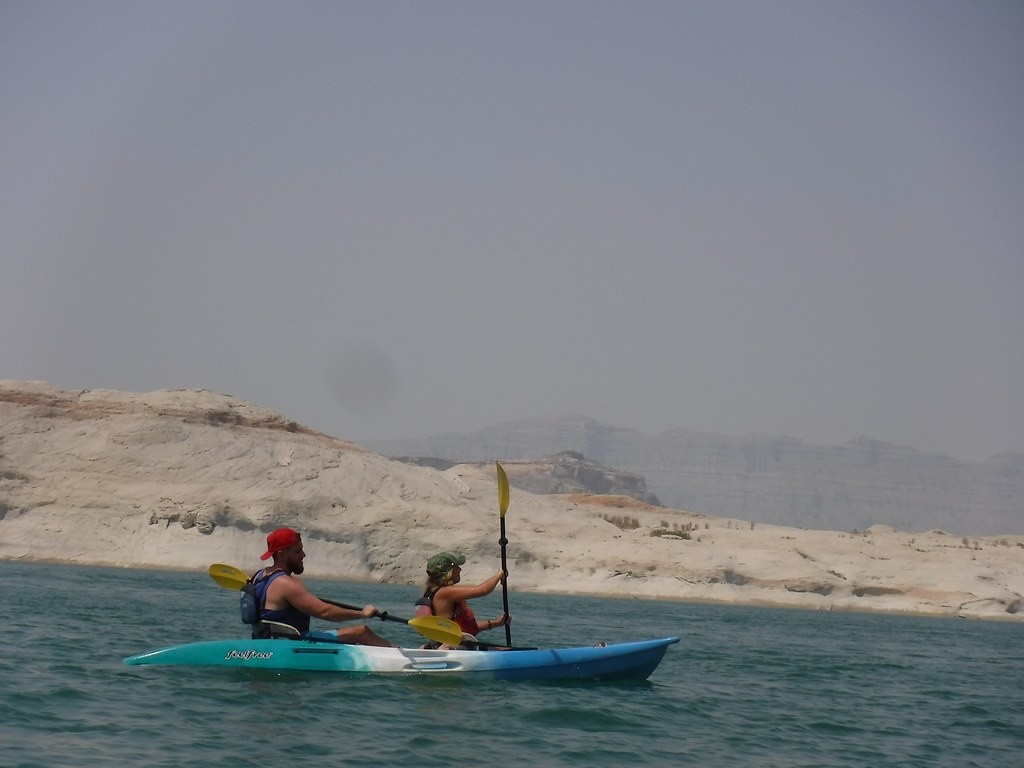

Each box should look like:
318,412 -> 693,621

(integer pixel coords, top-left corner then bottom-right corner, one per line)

494,458 -> 513,648
206,563 -> 464,649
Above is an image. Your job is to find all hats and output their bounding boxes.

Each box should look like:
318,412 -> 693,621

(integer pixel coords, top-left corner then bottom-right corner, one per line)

425,551 -> 466,577
260,528 -> 301,561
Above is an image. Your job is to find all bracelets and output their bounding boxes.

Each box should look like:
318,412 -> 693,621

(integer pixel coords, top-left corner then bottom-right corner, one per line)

488,620 -> 492,630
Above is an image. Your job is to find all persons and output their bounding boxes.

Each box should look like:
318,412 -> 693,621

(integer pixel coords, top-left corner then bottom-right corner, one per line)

249,528 -> 449,650
421,552 -> 607,651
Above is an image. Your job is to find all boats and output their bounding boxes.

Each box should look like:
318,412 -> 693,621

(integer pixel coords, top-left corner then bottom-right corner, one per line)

121,635 -> 682,680
